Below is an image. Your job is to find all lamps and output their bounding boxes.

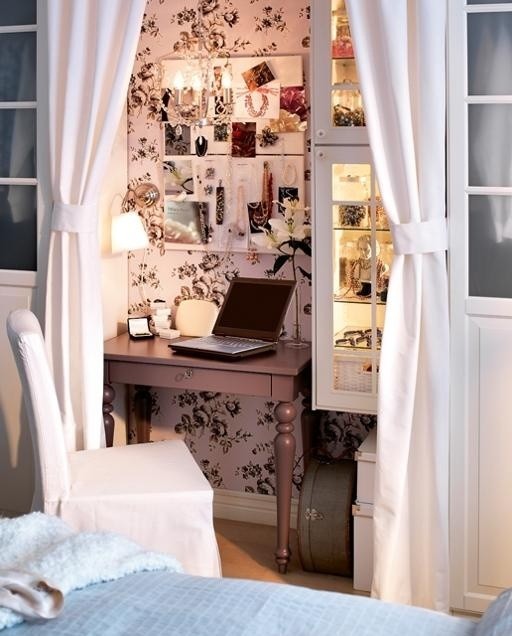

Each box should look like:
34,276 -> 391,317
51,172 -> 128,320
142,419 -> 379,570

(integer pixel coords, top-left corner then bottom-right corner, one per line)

150,0 -> 239,131
111,183 -> 159,254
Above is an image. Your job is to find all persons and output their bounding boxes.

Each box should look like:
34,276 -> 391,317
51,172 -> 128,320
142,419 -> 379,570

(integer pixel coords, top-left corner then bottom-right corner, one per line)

349,234 -> 390,283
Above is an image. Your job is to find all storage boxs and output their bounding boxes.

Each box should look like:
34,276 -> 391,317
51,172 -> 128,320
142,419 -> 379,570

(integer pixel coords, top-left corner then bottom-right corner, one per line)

296,425 -> 376,592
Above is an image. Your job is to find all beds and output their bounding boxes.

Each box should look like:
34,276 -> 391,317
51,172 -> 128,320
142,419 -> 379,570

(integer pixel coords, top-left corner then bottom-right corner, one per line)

0,510 -> 512,636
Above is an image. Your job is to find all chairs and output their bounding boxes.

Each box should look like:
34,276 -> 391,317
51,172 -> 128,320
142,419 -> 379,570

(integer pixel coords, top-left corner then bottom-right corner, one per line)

6,309 -> 221,577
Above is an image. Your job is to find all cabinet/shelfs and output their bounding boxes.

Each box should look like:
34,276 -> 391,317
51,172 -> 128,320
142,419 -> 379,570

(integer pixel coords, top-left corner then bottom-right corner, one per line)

312,1 -> 395,415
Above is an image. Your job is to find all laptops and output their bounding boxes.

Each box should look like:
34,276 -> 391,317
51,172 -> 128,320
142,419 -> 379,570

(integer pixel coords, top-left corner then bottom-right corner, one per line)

168,277 -> 297,360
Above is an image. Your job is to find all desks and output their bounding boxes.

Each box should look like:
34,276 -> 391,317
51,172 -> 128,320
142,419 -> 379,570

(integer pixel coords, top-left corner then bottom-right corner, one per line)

104,330 -> 320,575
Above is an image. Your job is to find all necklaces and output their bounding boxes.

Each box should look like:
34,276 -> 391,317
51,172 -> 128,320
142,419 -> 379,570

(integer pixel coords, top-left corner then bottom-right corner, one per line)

349,256 -> 384,282
163,57 -> 300,266
